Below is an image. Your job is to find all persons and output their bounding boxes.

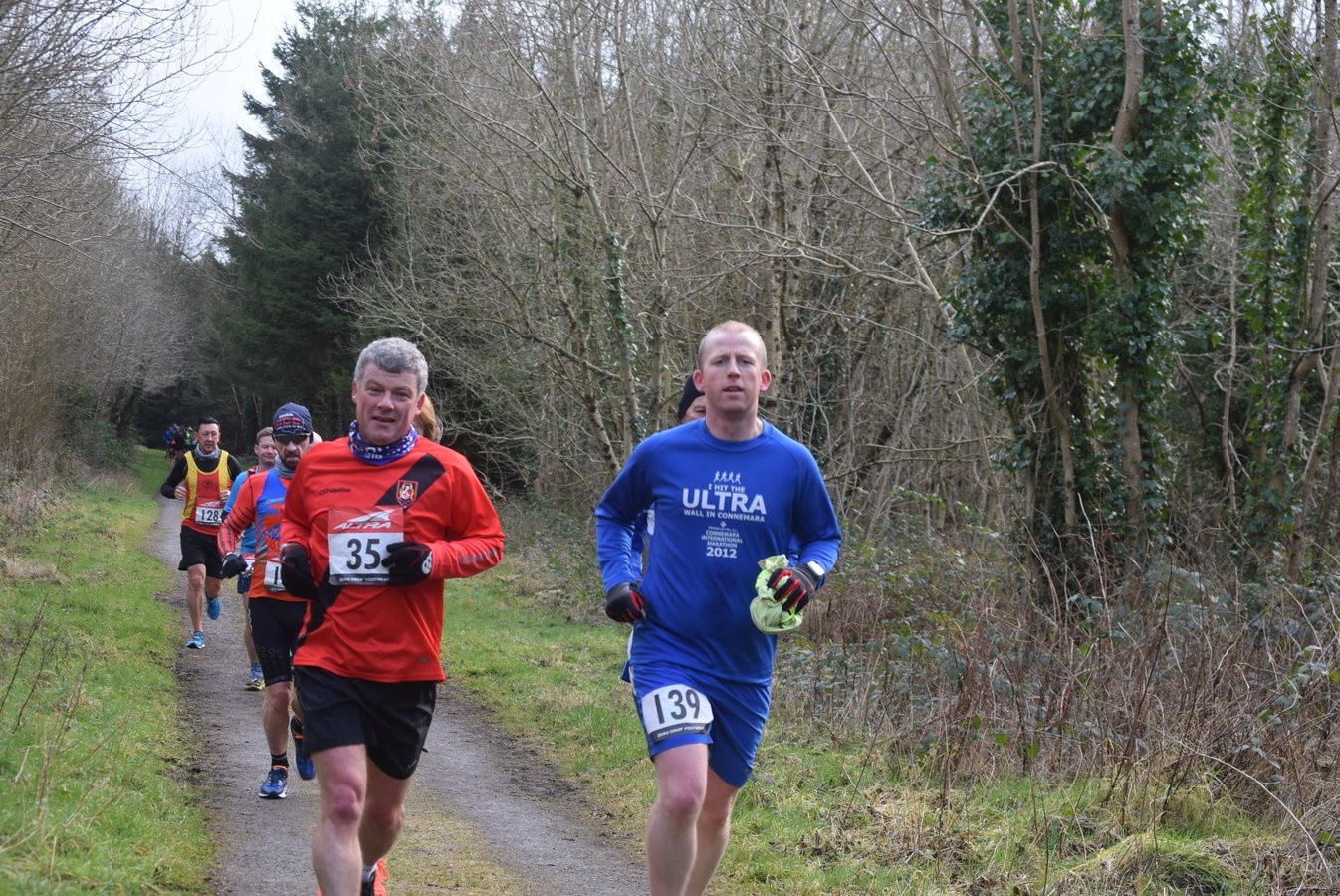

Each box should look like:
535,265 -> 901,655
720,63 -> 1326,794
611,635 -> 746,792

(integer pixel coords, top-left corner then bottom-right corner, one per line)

593,320 -> 845,896
162,423 -> 199,463
217,338 -> 505,894
630,374 -> 707,585
160,417 -> 243,650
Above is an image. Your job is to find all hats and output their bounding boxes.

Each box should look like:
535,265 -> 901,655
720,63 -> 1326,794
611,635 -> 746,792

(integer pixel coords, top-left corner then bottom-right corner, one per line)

679,373 -> 705,418
272,402 -> 312,435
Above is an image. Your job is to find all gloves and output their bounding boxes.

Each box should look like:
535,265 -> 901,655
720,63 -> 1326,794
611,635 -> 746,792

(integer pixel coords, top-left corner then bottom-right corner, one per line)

768,561 -> 828,614
604,584 -> 647,627
220,553 -> 248,579
280,542 -> 318,599
381,541 -> 433,585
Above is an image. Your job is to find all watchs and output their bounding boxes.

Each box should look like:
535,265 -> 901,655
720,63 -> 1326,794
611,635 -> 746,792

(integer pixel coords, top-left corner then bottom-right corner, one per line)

806,558 -> 825,579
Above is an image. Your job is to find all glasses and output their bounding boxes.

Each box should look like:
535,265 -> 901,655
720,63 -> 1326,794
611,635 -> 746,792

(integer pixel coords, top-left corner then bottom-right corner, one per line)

274,431 -> 311,445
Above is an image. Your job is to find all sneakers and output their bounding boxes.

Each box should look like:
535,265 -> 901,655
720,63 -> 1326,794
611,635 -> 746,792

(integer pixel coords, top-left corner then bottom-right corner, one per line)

186,632 -> 205,649
259,764 -> 287,798
290,717 -> 315,779
243,674 -> 266,690
204,591 -> 220,619
359,857 -> 392,895
